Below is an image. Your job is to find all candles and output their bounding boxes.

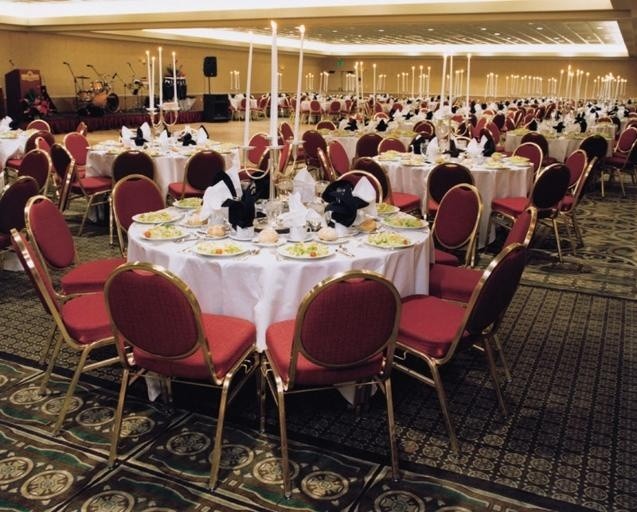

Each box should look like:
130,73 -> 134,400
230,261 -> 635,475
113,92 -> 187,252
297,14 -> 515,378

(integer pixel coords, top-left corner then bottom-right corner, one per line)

145,21 -> 304,147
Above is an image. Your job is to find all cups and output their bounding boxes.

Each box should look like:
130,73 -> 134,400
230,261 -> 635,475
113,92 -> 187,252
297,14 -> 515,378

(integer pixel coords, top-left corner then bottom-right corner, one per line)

286,218 -> 313,240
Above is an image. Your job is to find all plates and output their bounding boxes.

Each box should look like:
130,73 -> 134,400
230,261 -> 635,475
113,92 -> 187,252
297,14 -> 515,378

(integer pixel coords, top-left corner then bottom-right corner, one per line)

84,140 -> 238,159
131,197 -> 430,261
379,150 -> 533,171
